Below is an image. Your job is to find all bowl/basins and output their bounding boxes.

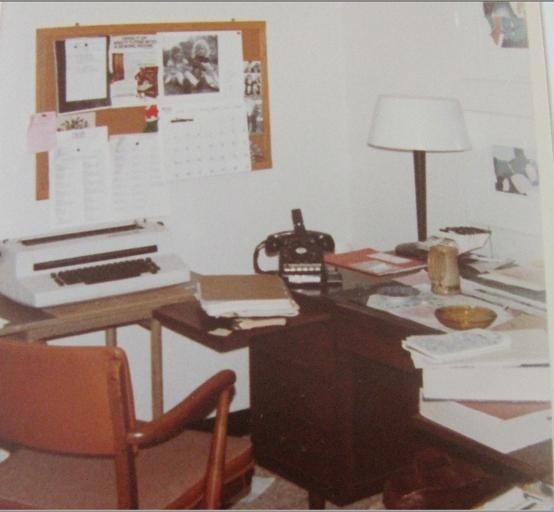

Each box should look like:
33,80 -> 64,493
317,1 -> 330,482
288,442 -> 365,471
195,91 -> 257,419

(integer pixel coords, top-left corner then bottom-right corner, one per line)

434,304 -> 497,329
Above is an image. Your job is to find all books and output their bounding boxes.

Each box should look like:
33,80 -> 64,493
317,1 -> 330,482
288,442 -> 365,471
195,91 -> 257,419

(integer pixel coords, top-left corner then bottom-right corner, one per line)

322,236 -> 553,455
194,270 -> 300,323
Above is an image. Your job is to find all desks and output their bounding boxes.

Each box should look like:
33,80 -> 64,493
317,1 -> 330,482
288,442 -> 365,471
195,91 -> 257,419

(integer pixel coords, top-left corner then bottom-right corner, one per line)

0,271 -> 211,423
150,244 -> 554,509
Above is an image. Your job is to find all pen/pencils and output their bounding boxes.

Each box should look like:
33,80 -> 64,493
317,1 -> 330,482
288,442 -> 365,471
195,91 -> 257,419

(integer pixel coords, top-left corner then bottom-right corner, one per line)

520,363 -> 550,367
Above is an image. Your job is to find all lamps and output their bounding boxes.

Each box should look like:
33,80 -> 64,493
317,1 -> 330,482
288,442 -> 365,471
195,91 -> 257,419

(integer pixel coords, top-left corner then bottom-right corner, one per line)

364,93 -> 474,245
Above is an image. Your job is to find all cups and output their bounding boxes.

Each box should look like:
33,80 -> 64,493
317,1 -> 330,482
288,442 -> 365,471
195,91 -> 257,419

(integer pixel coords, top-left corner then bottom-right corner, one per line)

426,244 -> 461,295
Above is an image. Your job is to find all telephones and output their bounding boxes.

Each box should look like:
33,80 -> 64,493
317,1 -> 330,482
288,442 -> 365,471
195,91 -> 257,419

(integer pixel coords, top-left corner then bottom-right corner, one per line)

265,231 -> 335,290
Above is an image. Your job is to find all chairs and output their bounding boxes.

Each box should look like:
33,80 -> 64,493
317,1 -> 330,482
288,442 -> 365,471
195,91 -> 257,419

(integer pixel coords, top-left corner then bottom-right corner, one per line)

0,335 -> 258,512
